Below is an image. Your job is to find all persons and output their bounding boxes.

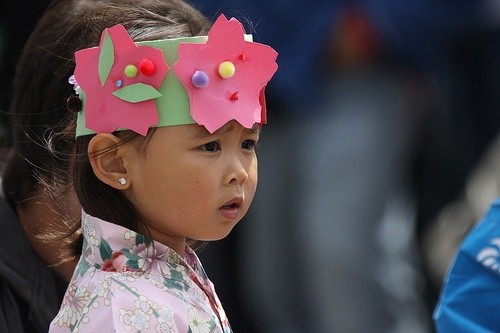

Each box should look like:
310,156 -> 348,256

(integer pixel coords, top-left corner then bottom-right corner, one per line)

0,0 -> 210,333
50,12 -> 279,332
